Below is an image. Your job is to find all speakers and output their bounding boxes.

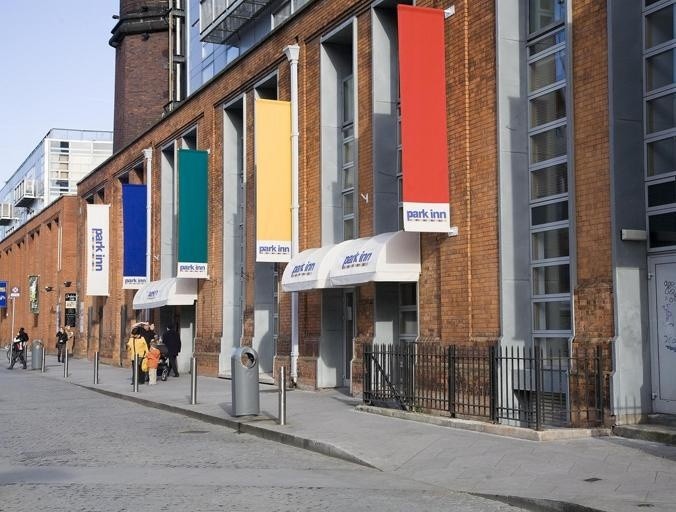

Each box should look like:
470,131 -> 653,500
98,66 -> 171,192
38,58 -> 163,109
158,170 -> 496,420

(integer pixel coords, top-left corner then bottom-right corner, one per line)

58,354 -> 64,363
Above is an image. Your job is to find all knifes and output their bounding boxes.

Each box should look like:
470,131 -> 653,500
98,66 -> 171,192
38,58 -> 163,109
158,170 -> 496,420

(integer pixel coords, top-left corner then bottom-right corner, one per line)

12,181 -> 35,202
2,202 -> 11,218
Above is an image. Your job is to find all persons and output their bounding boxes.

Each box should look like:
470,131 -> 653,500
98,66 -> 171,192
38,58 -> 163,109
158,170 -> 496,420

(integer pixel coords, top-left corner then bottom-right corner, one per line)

7,328 -> 28,370
126,322 -> 160,385
55,325 -> 74,363
162,325 -> 181,377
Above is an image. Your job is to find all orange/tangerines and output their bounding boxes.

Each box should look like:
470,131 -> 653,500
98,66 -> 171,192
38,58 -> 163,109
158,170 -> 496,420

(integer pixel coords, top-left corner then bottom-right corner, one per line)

144,342 -> 169,382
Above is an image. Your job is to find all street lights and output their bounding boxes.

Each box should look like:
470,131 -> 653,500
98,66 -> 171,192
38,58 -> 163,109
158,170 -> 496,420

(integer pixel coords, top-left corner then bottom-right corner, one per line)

138,357 -> 148,372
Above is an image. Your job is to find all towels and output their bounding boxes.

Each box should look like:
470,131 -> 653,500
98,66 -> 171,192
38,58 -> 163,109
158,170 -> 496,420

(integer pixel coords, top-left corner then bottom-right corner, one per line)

31,340 -> 43,369
230,347 -> 259,416
